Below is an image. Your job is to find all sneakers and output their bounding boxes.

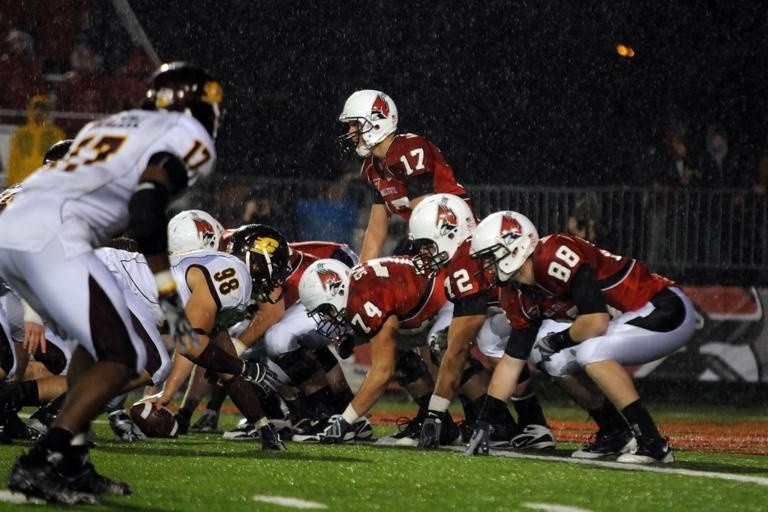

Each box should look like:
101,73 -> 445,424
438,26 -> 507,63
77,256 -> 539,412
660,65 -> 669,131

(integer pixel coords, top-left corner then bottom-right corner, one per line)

374,405 -> 464,447
569,430 -> 633,460
24,402 -> 99,442
5,442 -> 103,507
615,434 -> 676,465
0,381 -> 23,442
51,453 -> 134,501
458,418 -> 518,447
221,411 -> 295,440
491,422 -> 560,452
290,409 -> 373,444
190,408 -> 219,432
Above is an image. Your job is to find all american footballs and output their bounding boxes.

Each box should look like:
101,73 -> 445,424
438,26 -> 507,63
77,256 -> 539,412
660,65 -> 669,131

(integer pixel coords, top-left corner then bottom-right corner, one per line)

129,401 -> 180,438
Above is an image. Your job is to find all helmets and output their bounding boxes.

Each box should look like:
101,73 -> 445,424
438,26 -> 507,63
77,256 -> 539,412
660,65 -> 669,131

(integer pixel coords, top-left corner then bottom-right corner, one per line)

336,89 -> 401,160
224,222 -> 292,289
296,256 -> 355,324
142,59 -> 226,143
167,207 -> 225,260
42,137 -> 75,165
467,209 -> 540,288
407,190 -> 477,271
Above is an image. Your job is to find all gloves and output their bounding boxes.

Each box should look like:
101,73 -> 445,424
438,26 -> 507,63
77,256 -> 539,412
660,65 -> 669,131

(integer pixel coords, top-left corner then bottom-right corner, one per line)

252,415 -> 289,451
415,411 -> 445,452
154,288 -> 199,355
314,414 -> 355,444
333,333 -> 356,360
532,329 -> 563,364
107,406 -> 146,445
240,354 -> 294,398
462,416 -> 497,458
175,406 -> 192,435
